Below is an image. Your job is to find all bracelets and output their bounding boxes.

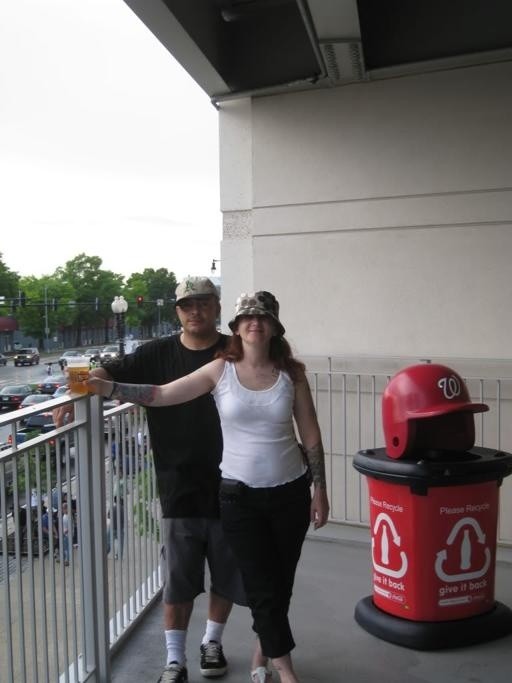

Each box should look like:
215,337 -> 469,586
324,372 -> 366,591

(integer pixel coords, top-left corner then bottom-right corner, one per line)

108,381 -> 116,401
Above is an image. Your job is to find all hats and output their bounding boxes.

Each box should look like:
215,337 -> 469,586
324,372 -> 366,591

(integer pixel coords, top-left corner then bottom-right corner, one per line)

228,290 -> 286,337
175,275 -> 220,303
46,508 -> 58,513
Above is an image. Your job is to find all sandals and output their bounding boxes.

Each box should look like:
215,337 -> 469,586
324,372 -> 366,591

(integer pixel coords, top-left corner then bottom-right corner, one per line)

251,666 -> 272,683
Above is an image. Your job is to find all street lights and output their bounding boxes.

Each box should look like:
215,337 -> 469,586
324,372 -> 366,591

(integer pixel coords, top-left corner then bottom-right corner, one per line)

111,294 -> 129,357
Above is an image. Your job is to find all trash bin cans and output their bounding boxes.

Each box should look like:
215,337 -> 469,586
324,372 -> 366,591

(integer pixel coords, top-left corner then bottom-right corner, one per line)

354,365 -> 512,651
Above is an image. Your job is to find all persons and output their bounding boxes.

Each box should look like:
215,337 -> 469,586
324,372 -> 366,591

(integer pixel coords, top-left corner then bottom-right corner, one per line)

47,362 -> 52,375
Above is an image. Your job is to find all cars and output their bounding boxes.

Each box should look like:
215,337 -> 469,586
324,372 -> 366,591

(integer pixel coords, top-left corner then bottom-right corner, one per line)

0,373 -> 130,436
1,353 -> 8,365
59,345 -> 118,370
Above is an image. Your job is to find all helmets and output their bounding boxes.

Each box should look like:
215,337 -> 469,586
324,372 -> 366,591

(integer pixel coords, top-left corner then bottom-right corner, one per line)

381,363 -> 490,459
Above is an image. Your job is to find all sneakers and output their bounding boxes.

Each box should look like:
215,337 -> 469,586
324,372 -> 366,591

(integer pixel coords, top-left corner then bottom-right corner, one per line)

157,660 -> 187,683
200,641 -> 228,678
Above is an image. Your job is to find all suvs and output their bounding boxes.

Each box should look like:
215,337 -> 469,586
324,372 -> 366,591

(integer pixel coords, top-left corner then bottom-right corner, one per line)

14,348 -> 40,366
